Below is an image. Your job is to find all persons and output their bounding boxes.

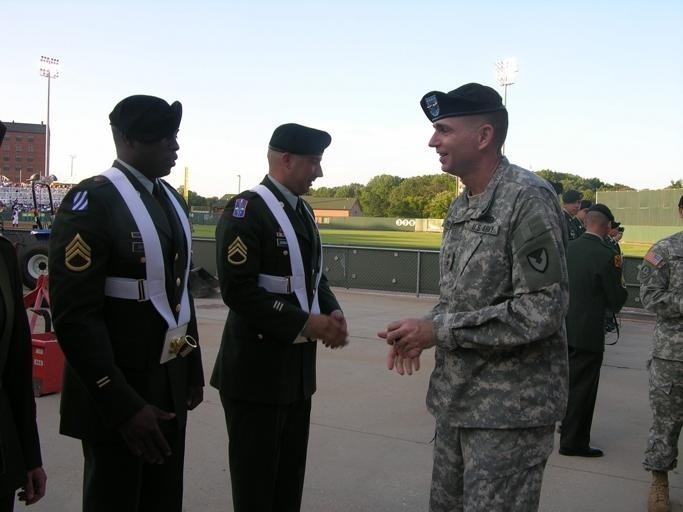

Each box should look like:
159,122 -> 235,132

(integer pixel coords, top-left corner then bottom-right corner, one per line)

374,82 -> 570,512
546,190 -> 631,457
210,123 -> 351,512
0,120 -> 47,512
636,194 -> 683,512
50,94 -> 206,512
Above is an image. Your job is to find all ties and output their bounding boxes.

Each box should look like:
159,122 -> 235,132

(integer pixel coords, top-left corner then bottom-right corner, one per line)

154,183 -> 166,213
296,198 -> 304,221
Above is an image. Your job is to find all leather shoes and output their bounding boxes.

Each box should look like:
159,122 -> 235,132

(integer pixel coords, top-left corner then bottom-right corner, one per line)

558,448 -> 602,457
606,320 -> 618,332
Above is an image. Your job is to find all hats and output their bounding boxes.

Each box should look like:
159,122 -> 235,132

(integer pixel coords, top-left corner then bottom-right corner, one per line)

420,82 -> 504,121
269,124 -> 330,156
109,96 -> 183,143
548,181 -> 623,233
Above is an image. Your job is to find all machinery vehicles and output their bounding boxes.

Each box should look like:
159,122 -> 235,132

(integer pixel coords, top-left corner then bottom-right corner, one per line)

17,182 -> 219,298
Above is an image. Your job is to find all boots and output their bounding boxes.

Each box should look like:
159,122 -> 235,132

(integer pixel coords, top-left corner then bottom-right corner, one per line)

647,471 -> 668,512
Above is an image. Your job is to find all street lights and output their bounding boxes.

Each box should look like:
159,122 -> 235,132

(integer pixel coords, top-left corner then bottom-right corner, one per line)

236,174 -> 241,195
493,55 -> 519,157
39,55 -> 59,179
